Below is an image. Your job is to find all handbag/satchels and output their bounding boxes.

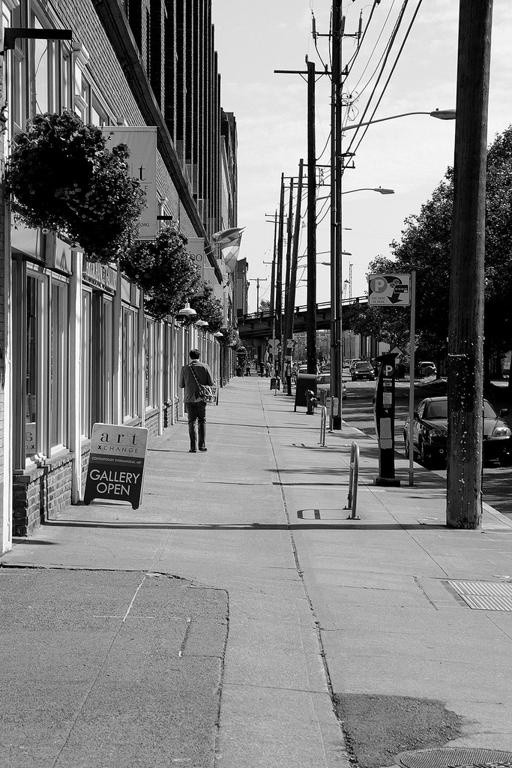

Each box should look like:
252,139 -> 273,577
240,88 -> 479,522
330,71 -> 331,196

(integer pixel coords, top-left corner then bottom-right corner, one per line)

199,384 -> 216,404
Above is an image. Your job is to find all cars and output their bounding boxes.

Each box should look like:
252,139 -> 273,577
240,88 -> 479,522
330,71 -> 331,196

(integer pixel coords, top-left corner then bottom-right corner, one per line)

343,358 -> 376,381
403,397 -> 512,465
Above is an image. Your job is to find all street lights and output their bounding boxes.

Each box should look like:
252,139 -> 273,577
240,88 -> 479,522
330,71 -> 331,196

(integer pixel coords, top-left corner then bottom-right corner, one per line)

330,108 -> 457,430
276,260 -> 330,390
281,251 -> 353,394
306,186 -> 395,375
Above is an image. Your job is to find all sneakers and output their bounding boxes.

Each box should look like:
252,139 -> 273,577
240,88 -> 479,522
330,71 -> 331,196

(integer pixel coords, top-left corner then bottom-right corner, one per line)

189,447 -> 207,453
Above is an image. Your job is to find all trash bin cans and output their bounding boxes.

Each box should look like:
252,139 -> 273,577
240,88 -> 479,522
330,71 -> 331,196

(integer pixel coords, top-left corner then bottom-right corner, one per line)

294,373 -> 317,412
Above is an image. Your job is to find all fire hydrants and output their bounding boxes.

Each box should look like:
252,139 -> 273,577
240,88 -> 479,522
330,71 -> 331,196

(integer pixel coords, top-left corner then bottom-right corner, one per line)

304,388 -> 318,415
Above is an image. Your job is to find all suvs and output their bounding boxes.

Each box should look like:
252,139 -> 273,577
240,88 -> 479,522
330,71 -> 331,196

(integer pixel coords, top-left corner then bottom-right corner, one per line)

417,361 -> 437,376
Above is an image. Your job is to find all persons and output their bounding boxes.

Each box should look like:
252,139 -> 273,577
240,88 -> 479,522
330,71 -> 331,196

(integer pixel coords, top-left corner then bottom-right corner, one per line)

235,349 -> 327,381
179,349 -> 213,452
